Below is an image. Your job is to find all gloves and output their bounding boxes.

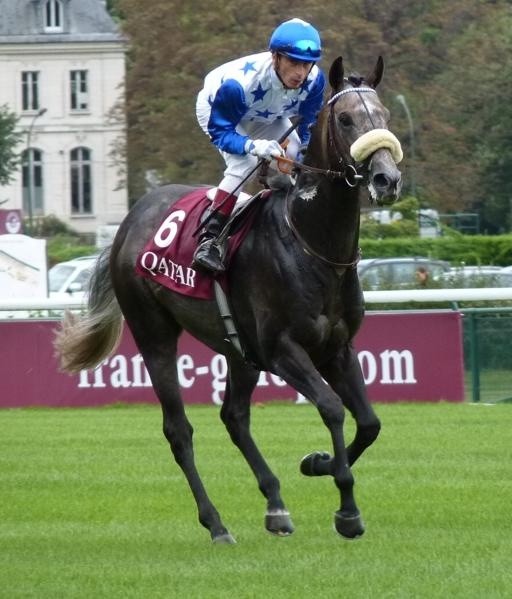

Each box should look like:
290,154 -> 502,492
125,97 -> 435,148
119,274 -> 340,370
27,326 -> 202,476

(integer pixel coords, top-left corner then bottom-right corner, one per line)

247,139 -> 286,160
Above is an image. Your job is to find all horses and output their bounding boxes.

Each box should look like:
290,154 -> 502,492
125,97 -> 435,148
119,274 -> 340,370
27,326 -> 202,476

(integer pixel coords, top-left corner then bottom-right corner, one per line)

51,54 -> 405,544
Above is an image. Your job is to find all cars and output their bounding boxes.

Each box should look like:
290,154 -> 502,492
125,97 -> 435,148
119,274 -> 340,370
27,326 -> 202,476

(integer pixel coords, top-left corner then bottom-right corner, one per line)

440,263 -> 511,288
48,255 -> 117,304
356,257 -> 452,289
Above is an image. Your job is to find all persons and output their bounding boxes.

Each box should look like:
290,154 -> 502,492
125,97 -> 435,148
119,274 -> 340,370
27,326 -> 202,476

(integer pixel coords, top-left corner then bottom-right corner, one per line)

190,16 -> 326,277
414,266 -> 429,286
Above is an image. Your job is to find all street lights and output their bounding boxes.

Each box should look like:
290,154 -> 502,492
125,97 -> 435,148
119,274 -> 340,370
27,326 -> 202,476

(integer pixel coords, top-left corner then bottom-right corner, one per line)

27,107 -> 47,235
394,92 -> 416,212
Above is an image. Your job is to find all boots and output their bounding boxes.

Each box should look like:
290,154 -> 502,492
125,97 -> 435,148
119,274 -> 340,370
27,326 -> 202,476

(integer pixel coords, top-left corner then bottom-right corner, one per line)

191,208 -> 229,274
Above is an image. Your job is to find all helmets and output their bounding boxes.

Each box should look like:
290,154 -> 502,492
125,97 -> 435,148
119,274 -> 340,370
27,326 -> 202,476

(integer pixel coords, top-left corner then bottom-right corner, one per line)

268,17 -> 323,63
